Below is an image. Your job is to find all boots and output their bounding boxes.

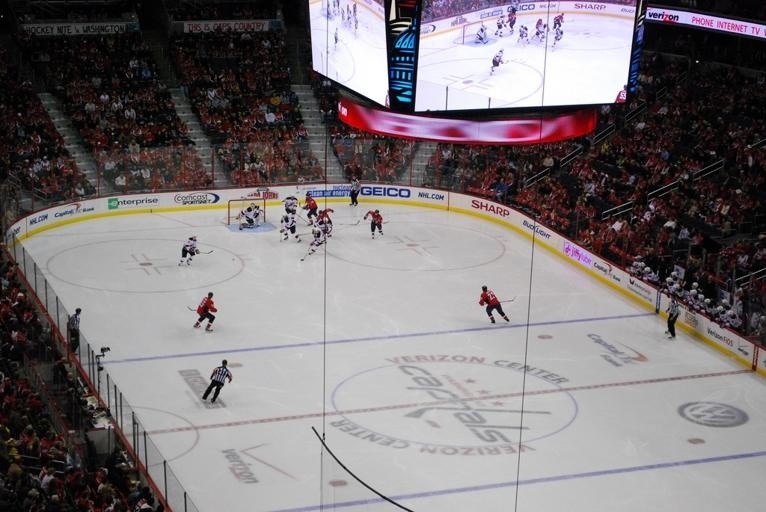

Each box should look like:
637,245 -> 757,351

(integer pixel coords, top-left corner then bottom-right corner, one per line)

504,316 -> 509,321
205,322 -> 213,331
193,321 -> 201,328
490,317 -> 495,323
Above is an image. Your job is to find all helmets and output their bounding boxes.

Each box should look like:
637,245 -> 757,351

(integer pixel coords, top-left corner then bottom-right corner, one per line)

282,197 -> 294,223
207,291 -> 214,297
633,256 -> 652,275
313,210 -> 329,239
482,285 -> 488,292
375,209 -> 379,213
665,271 -> 738,317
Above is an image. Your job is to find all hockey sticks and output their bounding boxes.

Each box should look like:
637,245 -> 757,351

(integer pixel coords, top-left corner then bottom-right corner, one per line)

334,220 -> 360,226
483,297 -> 516,305
197,249 -> 214,255
279,221 -> 283,243
364,215 -> 390,224
186,305 -> 197,311
301,243 -> 311,261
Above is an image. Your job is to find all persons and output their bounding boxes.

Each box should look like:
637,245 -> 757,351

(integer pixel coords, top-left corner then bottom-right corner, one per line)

559,234 -> 766,354
362,209 -> 385,240
203,358 -> 234,402
179,234 -> 200,266
307,226 -> 326,253
280,214 -> 302,242
478,286 -> 511,324
193,291 -> 218,331
